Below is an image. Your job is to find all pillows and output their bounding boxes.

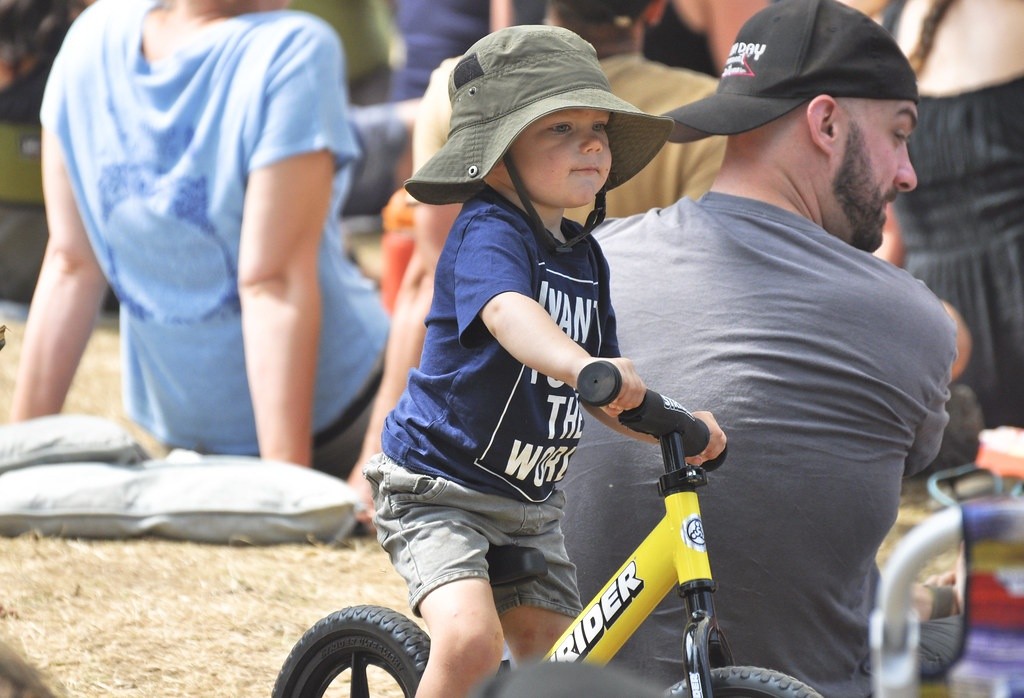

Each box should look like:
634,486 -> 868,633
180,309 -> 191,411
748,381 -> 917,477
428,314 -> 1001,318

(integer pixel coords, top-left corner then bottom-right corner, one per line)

0,417 -> 157,479
0,454 -> 363,545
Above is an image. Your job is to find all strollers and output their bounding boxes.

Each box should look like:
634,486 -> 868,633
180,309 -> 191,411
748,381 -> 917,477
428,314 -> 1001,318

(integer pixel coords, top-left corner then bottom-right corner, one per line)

863,455 -> 1022,698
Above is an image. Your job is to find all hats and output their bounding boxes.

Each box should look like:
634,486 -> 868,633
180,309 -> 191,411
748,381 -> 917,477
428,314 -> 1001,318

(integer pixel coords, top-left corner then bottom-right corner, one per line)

660,1 -> 919,144
403,25 -> 675,206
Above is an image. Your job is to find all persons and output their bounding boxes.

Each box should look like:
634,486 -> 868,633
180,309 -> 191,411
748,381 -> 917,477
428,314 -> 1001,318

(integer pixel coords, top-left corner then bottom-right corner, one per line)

10,0 -> 392,537
502,0 -> 959,698
290,1 -> 1024,485
345,0 -> 728,540
362,25 -> 726,698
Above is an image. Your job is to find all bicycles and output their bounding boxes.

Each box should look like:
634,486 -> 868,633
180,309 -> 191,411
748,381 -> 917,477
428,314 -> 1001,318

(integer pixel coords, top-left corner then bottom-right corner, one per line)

263,360 -> 815,698
